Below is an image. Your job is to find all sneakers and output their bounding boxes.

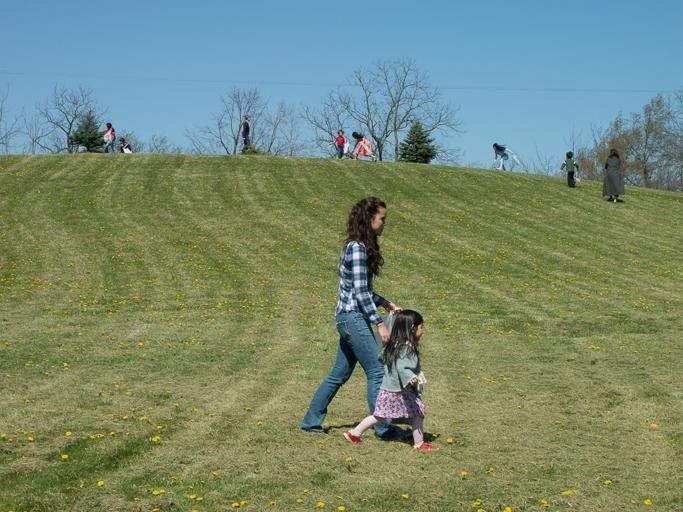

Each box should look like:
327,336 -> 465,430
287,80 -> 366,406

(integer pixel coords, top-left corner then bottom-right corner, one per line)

414,444 -> 440,454
343,430 -> 361,444
382,429 -> 411,442
299,424 -> 326,434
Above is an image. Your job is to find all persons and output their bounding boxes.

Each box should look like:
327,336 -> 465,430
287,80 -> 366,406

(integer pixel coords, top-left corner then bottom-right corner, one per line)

104,123 -> 116,153
242,115 -> 251,154
560,152 -> 578,188
602,149 -> 624,202
349,132 -> 377,162
493,143 -> 513,171
119,138 -> 131,153
300,197 -> 414,442
330,130 -> 347,161
343,310 -> 438,452
